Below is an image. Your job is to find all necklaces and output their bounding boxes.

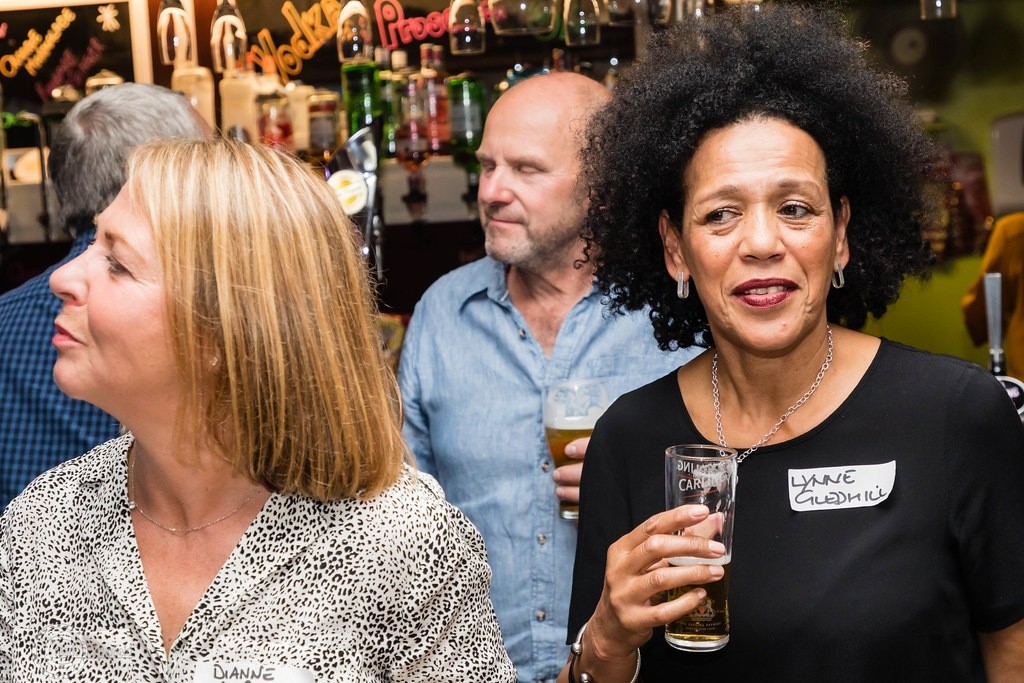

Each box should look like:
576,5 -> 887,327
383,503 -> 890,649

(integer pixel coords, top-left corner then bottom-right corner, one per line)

711,326 -> 833,496
129,455 -> 265,534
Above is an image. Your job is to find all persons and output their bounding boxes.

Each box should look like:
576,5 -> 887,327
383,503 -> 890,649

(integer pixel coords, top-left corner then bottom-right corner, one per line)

551,0 -> 1024,683
0,81 -> 215,515
397,67 -> 706,683
0,132 -> 518,683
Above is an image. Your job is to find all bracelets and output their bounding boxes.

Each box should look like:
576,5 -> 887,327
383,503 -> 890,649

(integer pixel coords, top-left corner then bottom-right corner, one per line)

568,622 -> 643,683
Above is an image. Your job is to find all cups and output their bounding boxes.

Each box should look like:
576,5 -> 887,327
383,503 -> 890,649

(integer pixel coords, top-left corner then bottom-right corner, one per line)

541,381 -> 610,519
448,0 -> 957,54
664,444 -> 738,651
336,0 -> 373,63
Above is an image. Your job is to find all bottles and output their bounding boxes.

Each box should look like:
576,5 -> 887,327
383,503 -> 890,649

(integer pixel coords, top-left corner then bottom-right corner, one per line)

255,93 -> 296,160
343,45 -> 484,169
306,90 -> 341,163
